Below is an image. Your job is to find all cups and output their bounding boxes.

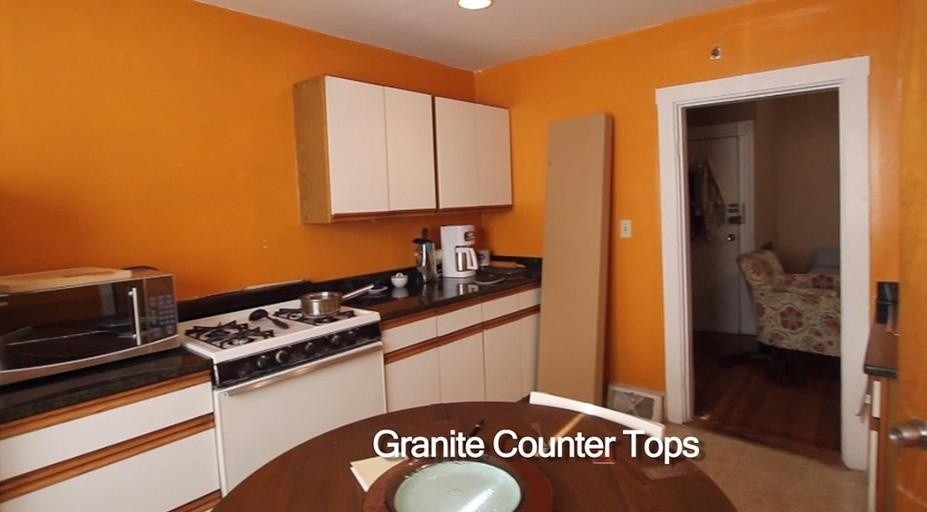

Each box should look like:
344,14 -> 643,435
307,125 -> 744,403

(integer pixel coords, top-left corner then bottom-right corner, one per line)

477,250 -> 491,267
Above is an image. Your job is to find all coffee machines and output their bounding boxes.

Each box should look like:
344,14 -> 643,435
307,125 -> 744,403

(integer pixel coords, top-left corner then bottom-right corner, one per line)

439,223 -> 480,279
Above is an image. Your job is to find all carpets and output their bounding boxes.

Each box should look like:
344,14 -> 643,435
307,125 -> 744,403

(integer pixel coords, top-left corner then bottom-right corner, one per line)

661,418 -> 870,511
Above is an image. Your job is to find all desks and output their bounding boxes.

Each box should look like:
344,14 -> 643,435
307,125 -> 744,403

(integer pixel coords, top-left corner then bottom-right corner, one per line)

206,398 -> 733,510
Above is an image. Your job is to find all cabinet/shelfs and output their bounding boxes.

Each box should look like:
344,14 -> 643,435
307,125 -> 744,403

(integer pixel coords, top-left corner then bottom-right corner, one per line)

362,295 -> 482,414
481,278 -> 542,403
433,97 -> 513,215
293,75 -> 435,226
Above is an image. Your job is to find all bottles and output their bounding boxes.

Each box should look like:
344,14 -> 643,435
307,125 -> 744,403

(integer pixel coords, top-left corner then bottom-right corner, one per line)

412,229 -> 437,285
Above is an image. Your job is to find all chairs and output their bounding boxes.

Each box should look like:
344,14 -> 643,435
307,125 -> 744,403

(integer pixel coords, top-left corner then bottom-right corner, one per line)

734,242 -> 840,361
529,392 -> 665,438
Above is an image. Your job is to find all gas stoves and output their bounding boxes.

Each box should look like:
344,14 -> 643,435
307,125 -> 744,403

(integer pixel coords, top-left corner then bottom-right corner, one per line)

175,293 -> 381,391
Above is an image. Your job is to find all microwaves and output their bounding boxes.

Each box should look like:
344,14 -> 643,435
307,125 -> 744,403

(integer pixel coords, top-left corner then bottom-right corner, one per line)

0,264 -> 182,393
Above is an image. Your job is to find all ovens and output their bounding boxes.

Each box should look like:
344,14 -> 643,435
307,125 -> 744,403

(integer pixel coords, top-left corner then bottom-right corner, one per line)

214,341 -> 385,500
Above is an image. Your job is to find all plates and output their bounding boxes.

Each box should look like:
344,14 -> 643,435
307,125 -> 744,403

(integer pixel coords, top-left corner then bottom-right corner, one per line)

361,448 -> 554,511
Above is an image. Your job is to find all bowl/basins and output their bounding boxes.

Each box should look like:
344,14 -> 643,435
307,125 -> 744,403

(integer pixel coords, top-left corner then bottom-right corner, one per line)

390,272 -> 408,289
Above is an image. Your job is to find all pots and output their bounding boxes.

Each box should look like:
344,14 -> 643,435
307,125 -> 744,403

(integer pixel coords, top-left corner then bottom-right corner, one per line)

300,283 -> 375,319
